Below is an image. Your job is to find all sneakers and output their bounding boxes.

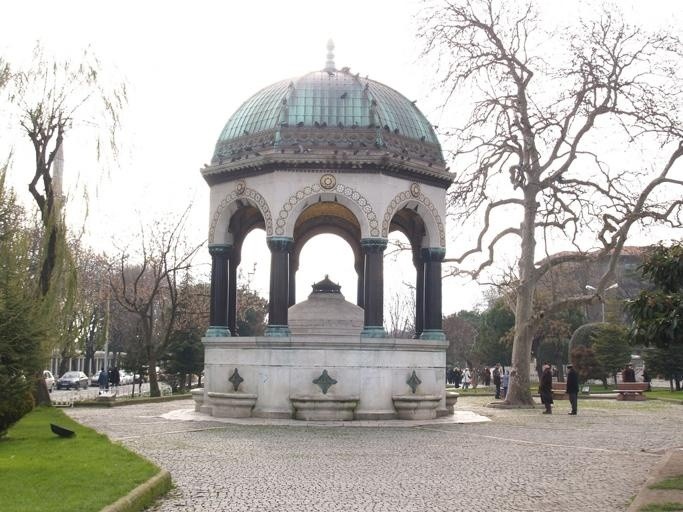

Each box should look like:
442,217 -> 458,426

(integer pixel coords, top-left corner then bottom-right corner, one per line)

569,412 -> 576,415
543,411 -> 551,414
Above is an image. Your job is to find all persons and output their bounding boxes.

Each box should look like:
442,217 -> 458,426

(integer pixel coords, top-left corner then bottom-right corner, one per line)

446,366 -> 490,389
96,367 -> 108,393
492,362 -> 501,399
621,364 -> 635,381
112,367 -> 119,386
502,369 -> 510,398
537,361 -> 552,413
565,365 -> 579,415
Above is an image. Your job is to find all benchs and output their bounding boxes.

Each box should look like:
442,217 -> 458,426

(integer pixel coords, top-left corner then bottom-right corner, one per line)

611,383 -> 649,401
551,383 -> 567,399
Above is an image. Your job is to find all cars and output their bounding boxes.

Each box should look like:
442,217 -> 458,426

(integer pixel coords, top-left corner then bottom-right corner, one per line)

40,365 -> 169,392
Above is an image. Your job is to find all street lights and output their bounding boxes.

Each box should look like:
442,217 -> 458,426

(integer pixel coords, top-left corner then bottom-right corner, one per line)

584,283 -> 620,323
